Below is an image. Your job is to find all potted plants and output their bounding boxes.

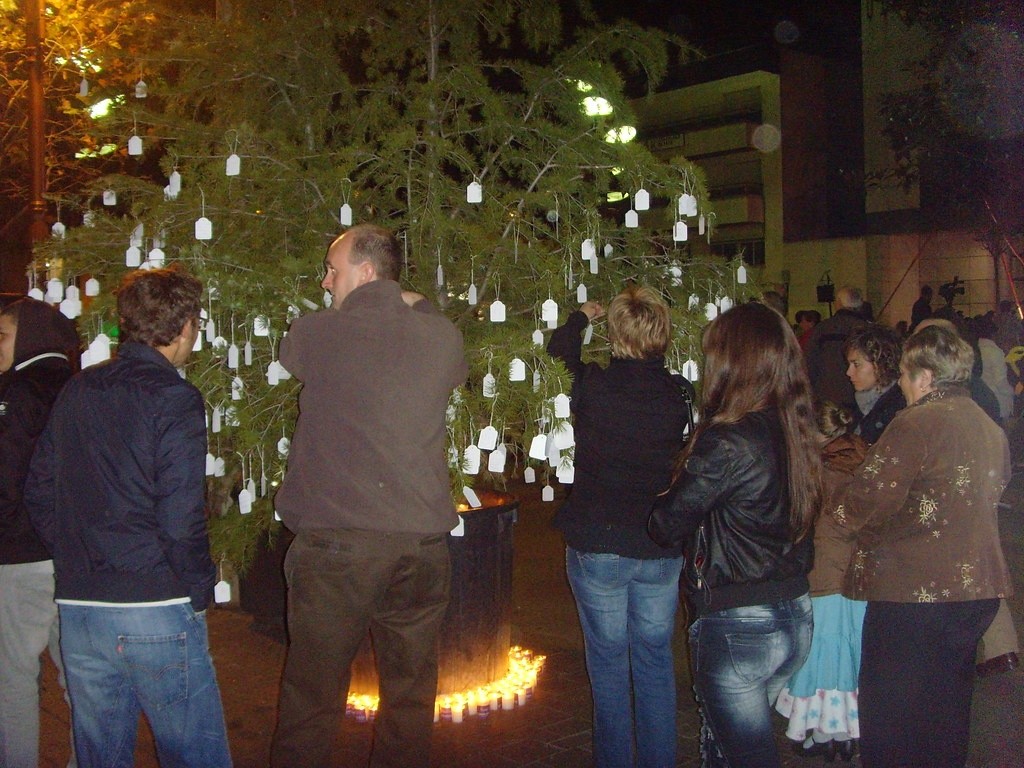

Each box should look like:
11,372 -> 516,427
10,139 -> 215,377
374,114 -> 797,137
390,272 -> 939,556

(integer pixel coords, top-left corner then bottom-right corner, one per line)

26,0 -> 764,708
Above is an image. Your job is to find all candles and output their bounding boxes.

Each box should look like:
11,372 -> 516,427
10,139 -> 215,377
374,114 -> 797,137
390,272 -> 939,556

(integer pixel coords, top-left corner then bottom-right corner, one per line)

346,644 -> 543,724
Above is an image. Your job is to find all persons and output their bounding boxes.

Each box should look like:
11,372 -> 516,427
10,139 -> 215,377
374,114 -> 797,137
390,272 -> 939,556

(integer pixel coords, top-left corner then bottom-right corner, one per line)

774,399 -> 869,761
0,297 -> 82,768
911,319 -> 1021,678
279,225 -> 466,721
647,302 -> 824,768
831,326 -> 1014,768
842,324 -> 907,446
23,269 -> 231,768
764,285 -> 875,405
547,285 -> 695,768
910,284 -> 1024,426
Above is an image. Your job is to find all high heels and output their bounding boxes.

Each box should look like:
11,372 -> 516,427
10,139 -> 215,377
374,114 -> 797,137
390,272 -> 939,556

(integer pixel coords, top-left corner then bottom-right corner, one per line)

792,736 -> 835,762
834,739 -> 856,761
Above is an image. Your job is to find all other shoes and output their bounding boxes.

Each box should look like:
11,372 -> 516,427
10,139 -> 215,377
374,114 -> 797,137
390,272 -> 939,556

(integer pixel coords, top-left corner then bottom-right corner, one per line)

976,651 -> 1020,678
